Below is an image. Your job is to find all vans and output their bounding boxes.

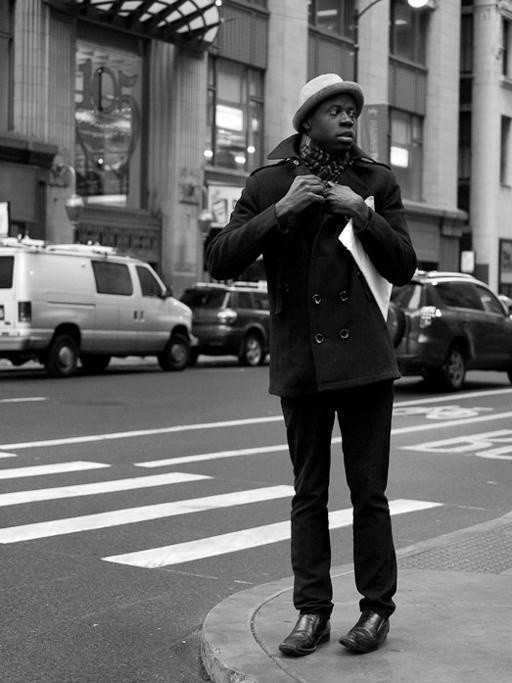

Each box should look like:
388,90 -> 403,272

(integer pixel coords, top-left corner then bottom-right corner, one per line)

0,231 -> 201,375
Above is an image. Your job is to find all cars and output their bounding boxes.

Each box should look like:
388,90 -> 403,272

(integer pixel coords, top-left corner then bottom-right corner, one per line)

481,292 -> 512,319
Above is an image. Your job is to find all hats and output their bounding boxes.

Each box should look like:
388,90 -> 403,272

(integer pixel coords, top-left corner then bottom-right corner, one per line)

292,72 -> 365,135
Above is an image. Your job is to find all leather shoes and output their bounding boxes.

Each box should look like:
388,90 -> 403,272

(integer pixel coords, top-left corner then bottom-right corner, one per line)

338,605 -> 390,652
279,613 -> 331,656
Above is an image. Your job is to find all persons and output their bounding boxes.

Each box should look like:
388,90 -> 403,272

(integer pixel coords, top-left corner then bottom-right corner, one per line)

206,72 -> 417,657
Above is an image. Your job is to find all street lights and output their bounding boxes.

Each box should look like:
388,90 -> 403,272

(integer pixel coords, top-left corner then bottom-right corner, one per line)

352,0 -> 429,85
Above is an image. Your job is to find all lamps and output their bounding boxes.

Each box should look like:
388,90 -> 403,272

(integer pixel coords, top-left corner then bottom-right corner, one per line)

48,164 -> 84,230
178,184 -> 216,242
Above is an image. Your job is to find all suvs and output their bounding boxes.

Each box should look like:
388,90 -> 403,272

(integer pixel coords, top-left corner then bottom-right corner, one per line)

383,264 -> 512,395
178,279 -> 272,368
